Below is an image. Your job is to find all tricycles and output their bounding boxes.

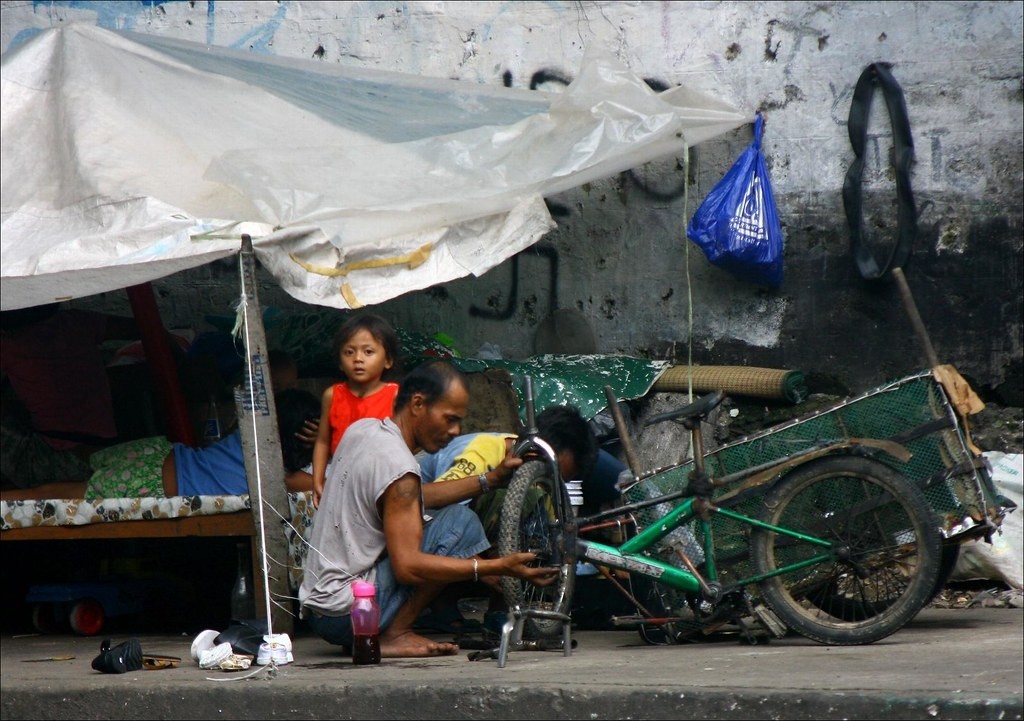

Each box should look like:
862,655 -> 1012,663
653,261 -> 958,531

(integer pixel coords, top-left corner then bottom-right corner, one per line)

479,372 -> 1019,677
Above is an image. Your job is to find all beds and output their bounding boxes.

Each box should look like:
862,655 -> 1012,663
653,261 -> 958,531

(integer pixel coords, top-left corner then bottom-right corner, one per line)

0,489 -> 316,640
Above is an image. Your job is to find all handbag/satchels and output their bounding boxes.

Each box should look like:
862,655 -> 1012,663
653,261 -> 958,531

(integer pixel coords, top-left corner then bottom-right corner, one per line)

686,115 -> 782,287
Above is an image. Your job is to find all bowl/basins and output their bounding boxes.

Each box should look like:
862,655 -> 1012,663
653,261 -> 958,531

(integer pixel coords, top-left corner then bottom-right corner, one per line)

575,564 -> 600,590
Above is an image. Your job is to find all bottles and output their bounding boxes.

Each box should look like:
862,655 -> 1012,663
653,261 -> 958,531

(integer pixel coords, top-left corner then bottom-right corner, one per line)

351,581 -> 381,664
231,542 -> 256,629
202,394 -> 223,448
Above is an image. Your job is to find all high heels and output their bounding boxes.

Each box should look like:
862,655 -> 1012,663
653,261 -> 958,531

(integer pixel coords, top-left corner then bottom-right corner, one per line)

213,615 -> 277,657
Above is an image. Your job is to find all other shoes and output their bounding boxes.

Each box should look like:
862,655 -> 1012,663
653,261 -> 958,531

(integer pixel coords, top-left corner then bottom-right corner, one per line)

92,639 -> 143,673
143,653 -> 183,670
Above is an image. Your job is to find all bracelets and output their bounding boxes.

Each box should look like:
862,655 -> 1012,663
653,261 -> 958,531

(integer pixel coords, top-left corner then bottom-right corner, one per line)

478,473 -> 489,495
473,559 -> 478,582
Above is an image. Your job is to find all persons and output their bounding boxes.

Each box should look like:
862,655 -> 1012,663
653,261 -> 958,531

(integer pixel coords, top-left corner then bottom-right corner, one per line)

0,387 -> 325,499
296,311 -> 669,657
